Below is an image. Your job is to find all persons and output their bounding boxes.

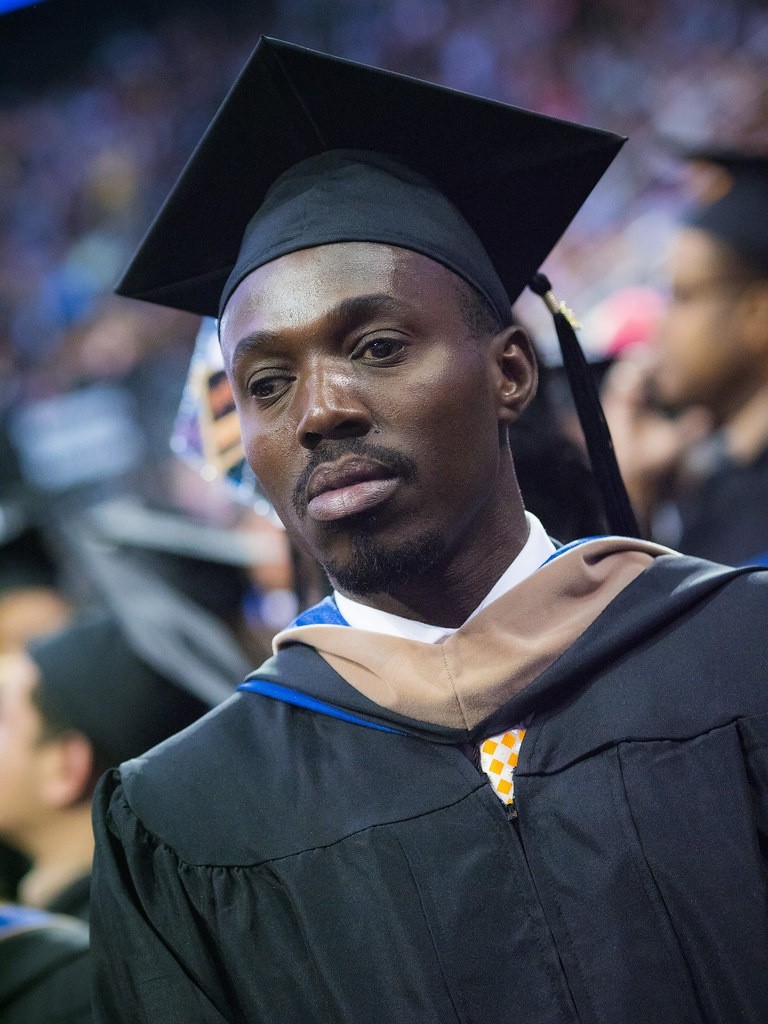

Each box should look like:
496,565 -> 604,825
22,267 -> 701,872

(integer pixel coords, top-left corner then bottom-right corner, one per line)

73,36 -> 768,1018
0,144 -> 765,1022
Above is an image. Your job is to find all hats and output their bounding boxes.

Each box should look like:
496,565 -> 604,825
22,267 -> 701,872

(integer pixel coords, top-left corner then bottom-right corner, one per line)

112,32 -> 641,539
